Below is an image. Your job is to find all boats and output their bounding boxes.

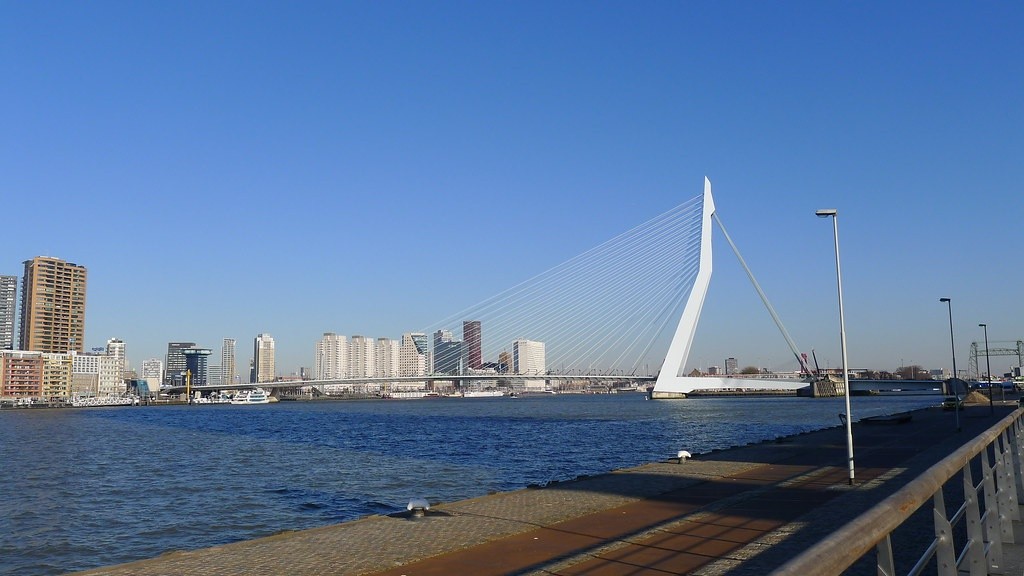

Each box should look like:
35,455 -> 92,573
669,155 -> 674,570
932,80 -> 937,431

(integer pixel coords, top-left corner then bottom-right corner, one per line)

188,391 -> 279,404
230,387 -> 269,404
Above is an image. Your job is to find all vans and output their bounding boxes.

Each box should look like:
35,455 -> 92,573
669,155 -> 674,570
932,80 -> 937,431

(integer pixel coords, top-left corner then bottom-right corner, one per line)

976,389 -> 990,398
1002,382 -> 1014,394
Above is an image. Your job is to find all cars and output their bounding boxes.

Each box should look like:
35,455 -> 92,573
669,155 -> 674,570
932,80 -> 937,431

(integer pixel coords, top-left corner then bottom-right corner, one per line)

941,396 -> 964,411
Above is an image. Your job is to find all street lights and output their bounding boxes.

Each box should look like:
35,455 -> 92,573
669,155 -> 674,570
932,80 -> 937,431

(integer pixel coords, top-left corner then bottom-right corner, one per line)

979,324 -> 995,415
939,297 -> 959,430
817,210 -> 855,484
